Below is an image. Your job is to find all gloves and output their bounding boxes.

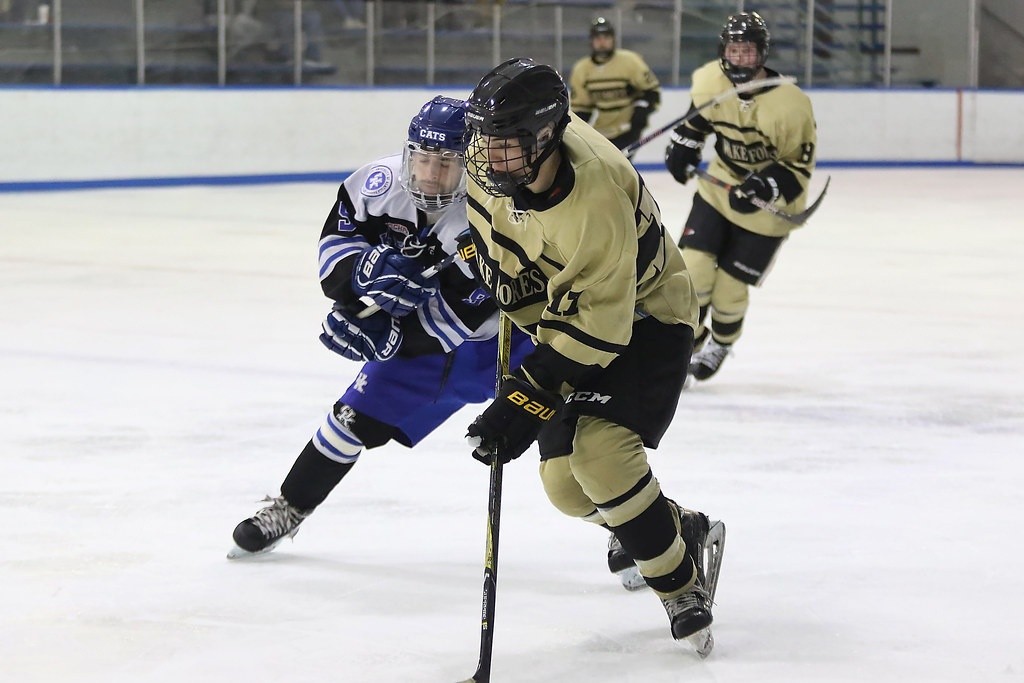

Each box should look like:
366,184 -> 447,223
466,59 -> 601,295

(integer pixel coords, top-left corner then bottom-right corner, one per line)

319,301 -> 405,363
351,244 -> 435,316
728,160 -> 803,214
455,229 -> 483,285
664,124 -> 707,184
463,378 -> 564,465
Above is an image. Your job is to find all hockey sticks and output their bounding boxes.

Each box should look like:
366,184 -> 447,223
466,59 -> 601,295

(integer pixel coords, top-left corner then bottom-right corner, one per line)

454,306 -> 513,683
355,76 -> 799,318
686,162 -> 830,225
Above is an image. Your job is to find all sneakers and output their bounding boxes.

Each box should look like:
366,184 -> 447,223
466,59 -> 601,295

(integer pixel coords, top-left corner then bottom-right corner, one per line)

608,532 -> 646,590
226,495 -> 312,560
666,495 -> 727,607
660,576 -> 718,658
687,339 -> 733,389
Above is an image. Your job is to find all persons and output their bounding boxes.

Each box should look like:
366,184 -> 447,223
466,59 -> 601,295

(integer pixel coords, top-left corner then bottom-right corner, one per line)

262,0 -> 365,60
226,95 -> 537,561
463,57 -> 725,657
567,16 -> 661,162
666,11 -> 817,385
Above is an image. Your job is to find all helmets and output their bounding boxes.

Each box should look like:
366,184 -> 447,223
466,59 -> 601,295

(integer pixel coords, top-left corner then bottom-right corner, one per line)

408,95 -> 478,159
589,18 -> 614,57
718,12 -> 770,82
461,57 -> 571,198
